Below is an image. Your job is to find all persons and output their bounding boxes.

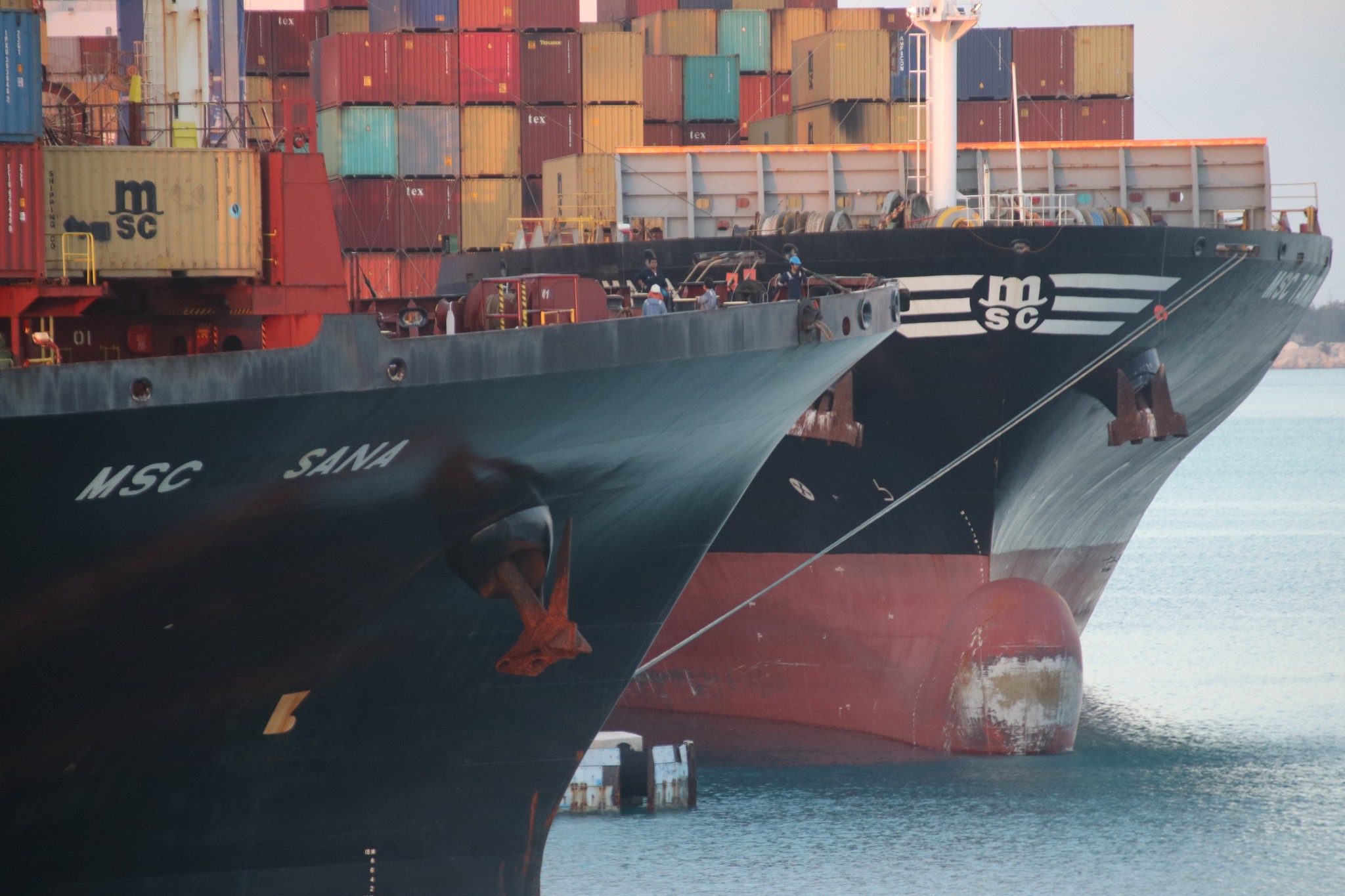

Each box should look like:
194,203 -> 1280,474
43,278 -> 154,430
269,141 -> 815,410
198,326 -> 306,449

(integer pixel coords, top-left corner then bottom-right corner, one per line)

771,256 -> 808,300
634,249 -> 671,297
695,278 -> 717,311
640,284 -> 667,316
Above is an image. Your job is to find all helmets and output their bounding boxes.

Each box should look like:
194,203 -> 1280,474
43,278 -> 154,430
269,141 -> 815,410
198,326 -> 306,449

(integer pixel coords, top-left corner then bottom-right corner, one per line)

790,256 -> 802,265
651,283 -> 661,294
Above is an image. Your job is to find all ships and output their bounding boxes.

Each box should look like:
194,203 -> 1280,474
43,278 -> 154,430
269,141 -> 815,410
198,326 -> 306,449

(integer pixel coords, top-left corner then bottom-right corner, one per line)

1,2 -> 911,896
435,0 -> 1333,896
